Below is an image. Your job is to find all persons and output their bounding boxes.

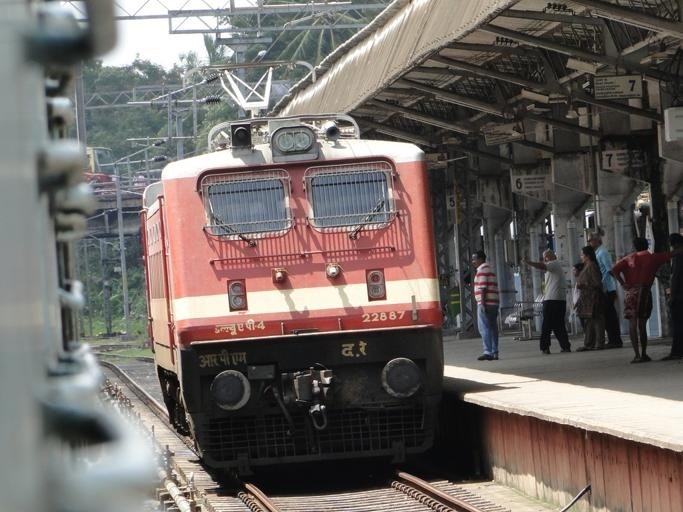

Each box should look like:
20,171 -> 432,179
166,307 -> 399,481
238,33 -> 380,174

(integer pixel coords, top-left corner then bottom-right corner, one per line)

471,250 -> 500,360
573,232 -> 623,351
661,232 -> 683,361
610,237 -> 682,363
523,249 -> 571,354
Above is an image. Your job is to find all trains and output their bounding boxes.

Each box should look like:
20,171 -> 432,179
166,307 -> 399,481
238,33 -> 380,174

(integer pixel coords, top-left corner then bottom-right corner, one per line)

140,112 -> 445,482
86,147 -> 120,183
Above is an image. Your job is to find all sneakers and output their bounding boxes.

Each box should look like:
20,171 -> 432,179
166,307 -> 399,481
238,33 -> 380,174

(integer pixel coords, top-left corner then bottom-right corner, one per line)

477,351 -> 499,361
538,339 -> 625,356
630,354 -> 652,364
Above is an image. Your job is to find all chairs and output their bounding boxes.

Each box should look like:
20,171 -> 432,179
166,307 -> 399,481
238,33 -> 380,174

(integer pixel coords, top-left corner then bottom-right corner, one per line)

511,292 -> 544,342
